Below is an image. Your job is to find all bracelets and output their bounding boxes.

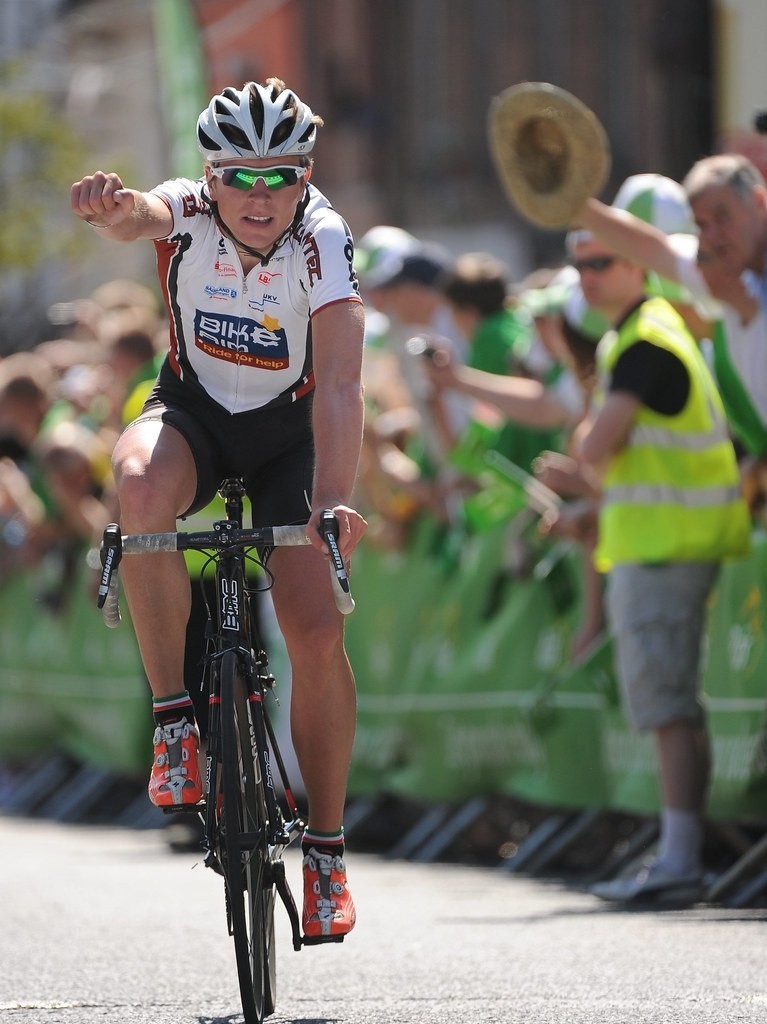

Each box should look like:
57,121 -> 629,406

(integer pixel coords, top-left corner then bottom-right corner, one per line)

86,221 -> 111,228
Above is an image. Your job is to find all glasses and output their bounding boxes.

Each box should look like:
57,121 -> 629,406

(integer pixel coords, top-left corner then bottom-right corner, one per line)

570,254 -> 623,272
210,165 -> 309,192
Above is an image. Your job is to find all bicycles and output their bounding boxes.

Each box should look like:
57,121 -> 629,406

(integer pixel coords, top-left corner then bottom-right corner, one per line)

97,467 -> 356,1024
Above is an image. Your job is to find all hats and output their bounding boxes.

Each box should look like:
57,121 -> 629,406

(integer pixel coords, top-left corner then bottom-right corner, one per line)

611,173 -> 703,236
353,225 -> 423,293
483,79 -> 613,228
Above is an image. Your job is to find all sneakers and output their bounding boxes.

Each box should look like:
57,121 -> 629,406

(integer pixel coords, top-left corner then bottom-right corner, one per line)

148,716 -> 205,809
301,848 -> 356,938
585,857 -> 708,905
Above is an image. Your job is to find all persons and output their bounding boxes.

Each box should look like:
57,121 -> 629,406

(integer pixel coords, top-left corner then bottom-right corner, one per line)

358,111 -> 767,907
0,282 -> 173,599
70,80 -> 365,936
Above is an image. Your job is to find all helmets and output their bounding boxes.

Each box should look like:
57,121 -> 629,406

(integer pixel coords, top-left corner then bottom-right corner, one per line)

195,81 -> 319,162
